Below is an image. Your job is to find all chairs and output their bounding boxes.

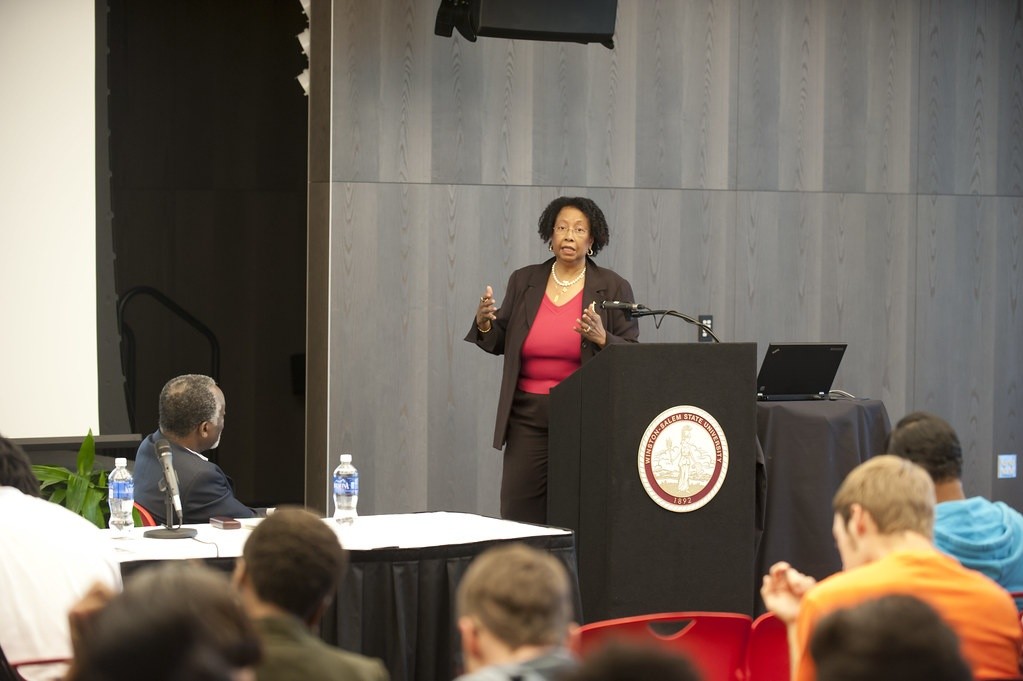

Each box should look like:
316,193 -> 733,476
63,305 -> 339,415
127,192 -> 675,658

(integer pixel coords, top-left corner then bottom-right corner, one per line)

576,608 -> 752,681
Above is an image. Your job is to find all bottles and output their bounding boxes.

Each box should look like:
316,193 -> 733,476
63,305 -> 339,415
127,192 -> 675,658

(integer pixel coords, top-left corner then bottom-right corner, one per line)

331,454 -> 360,529
107,458 -> 135,538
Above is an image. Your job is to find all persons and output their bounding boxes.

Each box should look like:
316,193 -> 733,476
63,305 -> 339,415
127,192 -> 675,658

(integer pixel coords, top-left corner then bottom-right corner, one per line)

464,197 -> 640,525
795,592 -> 975,681
0,435 -> 125,681
0,584 -> 116,681
132,374 -> 266,525
575,646 -> 706,681
229,509 -> 388,681
82,558 -> 264,681
452,543 -> 581,681
760,454 -> 1023,681
887,412 -> 1023,609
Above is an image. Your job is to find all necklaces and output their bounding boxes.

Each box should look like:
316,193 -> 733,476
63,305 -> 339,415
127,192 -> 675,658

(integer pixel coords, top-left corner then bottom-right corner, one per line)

552,261 -> 586,302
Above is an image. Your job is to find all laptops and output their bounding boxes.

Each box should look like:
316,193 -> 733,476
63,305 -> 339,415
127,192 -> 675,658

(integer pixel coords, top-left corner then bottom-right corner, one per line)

757,342 -> 848,402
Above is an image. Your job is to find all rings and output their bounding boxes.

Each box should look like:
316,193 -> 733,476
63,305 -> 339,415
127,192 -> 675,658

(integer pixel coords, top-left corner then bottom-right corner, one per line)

583,327 -> 590,332
480,296 -> 488,303
478,326 -> 491,333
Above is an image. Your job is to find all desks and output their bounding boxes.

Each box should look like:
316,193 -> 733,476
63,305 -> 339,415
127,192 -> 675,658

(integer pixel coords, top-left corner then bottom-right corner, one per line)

176,506 -> 576,681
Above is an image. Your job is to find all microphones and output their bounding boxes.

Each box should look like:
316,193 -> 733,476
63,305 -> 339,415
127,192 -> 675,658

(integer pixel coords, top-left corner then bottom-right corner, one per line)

155,439 -> 183,520
600,300 -> 645,310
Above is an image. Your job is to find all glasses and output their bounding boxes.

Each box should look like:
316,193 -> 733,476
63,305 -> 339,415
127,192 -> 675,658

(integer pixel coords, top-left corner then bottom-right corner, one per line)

553,225 -> 591,236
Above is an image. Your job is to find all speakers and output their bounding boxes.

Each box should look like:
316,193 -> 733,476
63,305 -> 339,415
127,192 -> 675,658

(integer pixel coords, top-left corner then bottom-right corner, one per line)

470,0 -> 618,43
